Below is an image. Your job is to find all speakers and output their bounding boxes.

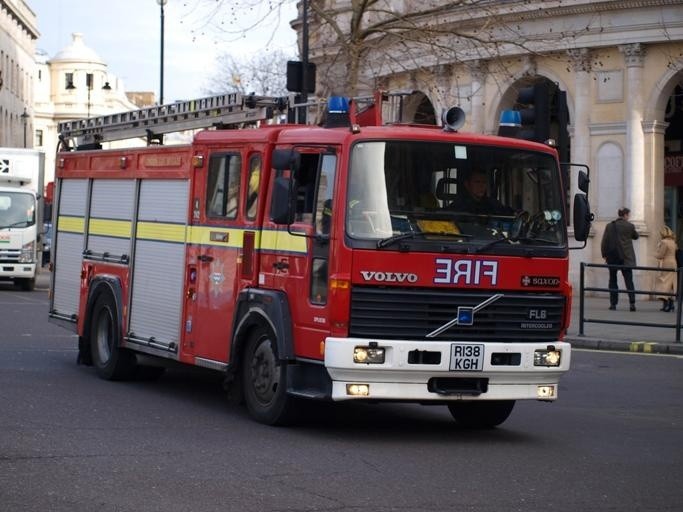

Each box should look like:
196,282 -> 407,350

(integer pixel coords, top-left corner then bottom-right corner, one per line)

286,60 -> 316,93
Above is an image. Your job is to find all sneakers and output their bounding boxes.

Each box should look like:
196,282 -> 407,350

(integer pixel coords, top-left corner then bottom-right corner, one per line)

608,303 -> 636,311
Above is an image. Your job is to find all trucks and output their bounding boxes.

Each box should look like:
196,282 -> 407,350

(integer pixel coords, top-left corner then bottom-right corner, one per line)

0,148 -> 54,291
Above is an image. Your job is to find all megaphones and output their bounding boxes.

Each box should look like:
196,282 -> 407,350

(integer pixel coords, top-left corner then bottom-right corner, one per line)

440,107 -> 466,133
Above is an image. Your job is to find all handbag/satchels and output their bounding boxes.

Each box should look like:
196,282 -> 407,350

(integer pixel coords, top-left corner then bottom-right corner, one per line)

605,241 -> 626,271
675,248 -> 683,268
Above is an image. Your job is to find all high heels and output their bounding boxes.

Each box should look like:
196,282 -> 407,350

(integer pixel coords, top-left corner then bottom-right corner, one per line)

659,300 -> 674,312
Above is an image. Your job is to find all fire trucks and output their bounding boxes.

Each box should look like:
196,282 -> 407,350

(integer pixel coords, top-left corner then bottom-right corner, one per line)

46,92 -> 595,427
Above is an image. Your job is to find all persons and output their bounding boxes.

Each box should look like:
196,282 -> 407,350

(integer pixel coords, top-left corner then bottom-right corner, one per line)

444,164 -> 529,229
652,225 -> 677,313
600,206 -> 639,311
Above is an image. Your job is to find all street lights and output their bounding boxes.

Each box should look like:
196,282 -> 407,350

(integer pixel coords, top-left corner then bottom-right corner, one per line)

156,0 -> 166,105
21,108 -> 31,148
